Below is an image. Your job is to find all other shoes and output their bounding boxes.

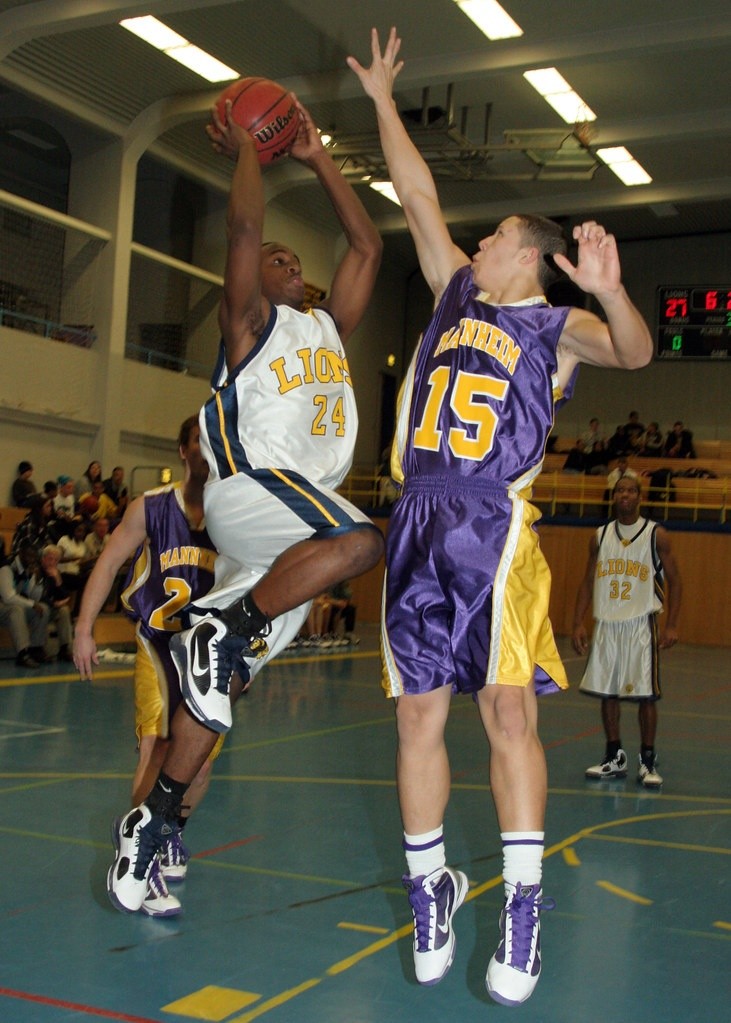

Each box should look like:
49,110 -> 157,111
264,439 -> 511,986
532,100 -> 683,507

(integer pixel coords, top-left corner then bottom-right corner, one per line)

57,646 -> 73,662
15,655 -> 40,668
33,646 -> 52,664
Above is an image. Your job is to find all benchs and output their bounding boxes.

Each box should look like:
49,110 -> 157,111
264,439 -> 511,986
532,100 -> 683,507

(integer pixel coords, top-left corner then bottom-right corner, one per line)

0,504 -> 137,653
533,436 -> 731,527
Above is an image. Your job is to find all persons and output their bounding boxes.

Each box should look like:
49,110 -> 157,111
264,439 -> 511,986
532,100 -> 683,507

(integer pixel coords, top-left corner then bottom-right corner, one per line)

69,414 -> 227,880
562,411 -> 696,502
0,461 -> 138,668
344,27 -> 653,1010
287,580 -> 360,649
378,439 -> 401,517
105,90 -> 382,919
570,475 -> 680,787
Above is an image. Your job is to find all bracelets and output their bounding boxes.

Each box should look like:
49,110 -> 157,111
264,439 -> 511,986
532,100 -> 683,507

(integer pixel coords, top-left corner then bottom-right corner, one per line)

666,622 -> 677,628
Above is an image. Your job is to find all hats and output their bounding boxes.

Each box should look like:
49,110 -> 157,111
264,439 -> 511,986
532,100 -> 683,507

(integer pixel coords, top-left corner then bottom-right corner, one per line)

19,461 -> 32,475
44,481 -> 58,493
57,475 -> 73,488
26,492 -> 49,510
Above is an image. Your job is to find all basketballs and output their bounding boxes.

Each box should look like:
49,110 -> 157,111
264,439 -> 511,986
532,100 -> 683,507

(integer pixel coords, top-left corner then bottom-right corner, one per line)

214,78 -> 301,164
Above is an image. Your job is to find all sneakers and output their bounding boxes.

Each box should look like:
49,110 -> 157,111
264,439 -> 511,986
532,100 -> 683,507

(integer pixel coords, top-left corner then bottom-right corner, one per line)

285,631 -> 360,651
400,865 -> 469,985
155,812 -> 190,881
482,878 -> 554,1003
105,788 -> 181,916
168,593 -> 276,736
142,856 -> 179,916
636,752 -> 662,789
585,746 -> 627,779
